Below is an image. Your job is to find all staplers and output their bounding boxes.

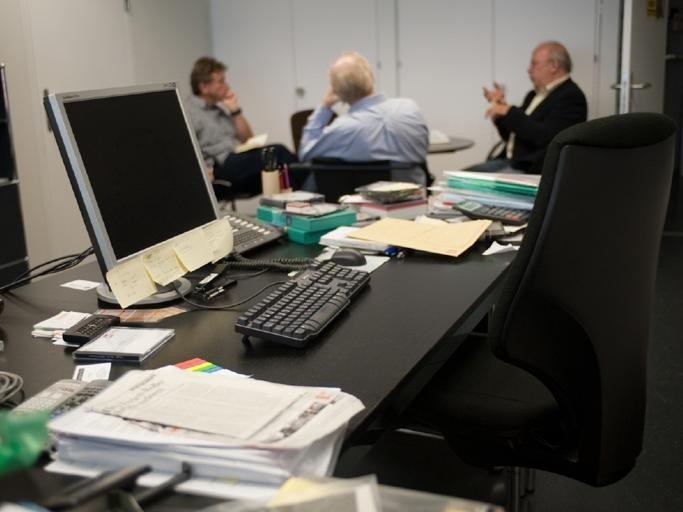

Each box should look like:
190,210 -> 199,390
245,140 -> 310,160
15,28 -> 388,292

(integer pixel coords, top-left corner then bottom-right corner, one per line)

191,263 -> 238,301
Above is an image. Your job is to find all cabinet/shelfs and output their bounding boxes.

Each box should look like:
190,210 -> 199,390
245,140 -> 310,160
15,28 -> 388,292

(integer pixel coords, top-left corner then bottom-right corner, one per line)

1,64 -> 31,290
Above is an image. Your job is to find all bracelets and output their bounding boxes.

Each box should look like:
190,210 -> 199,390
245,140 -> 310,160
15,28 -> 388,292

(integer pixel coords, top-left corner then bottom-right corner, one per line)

229,108 -> 241,116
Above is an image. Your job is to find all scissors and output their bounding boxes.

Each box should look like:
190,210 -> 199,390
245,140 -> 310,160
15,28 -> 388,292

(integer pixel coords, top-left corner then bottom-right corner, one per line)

260,145 -> 279,171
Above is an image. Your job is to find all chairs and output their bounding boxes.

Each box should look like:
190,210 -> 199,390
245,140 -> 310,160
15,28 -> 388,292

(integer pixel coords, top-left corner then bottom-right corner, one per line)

308,152 -> 396,204
290,109 -> 339,153
382,107 -> 682,511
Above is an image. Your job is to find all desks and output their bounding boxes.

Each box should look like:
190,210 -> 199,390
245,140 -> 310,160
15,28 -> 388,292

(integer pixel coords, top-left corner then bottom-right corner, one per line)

290,129 -> 477,167
0,180 -> 528,512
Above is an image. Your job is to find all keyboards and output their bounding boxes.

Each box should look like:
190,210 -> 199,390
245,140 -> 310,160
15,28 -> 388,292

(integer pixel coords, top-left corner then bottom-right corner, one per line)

234,261 -> 371,349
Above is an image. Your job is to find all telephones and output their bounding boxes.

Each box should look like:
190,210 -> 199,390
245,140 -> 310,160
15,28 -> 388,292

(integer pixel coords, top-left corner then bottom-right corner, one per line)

221,212 -> 282,260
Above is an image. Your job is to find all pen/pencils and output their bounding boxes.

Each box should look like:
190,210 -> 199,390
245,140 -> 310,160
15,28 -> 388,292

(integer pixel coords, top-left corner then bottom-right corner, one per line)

277,163 -> 290,193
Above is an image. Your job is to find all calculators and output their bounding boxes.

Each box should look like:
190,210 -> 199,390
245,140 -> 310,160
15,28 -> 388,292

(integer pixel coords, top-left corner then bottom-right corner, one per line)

452,199 -> 532,226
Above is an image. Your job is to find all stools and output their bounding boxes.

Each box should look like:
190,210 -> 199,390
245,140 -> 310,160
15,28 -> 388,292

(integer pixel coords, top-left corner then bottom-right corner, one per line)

209,174 -> 264,215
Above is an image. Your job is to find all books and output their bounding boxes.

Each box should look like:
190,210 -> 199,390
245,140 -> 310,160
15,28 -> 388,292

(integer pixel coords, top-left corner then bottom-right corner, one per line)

318,165 -> 547,255
283,199 -> 341,216
44,358 -> 369,499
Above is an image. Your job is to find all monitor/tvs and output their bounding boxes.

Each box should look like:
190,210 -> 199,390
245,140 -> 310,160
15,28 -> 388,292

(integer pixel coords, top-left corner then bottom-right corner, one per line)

43,81 -> 223,309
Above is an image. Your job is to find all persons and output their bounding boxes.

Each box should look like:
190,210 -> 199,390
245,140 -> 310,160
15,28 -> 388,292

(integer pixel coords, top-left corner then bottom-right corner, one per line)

481,40 -> 588,174
298,51 -> 429,186
180,56 -> 295,201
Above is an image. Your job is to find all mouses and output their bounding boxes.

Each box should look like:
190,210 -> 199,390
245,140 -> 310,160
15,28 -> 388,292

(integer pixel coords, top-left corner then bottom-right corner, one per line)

322,248 -> 366,266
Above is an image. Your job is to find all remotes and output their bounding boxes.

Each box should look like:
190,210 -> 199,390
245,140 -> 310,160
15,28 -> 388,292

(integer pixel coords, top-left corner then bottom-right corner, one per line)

47,380 -> 112,421
7,380 -> 86,425
